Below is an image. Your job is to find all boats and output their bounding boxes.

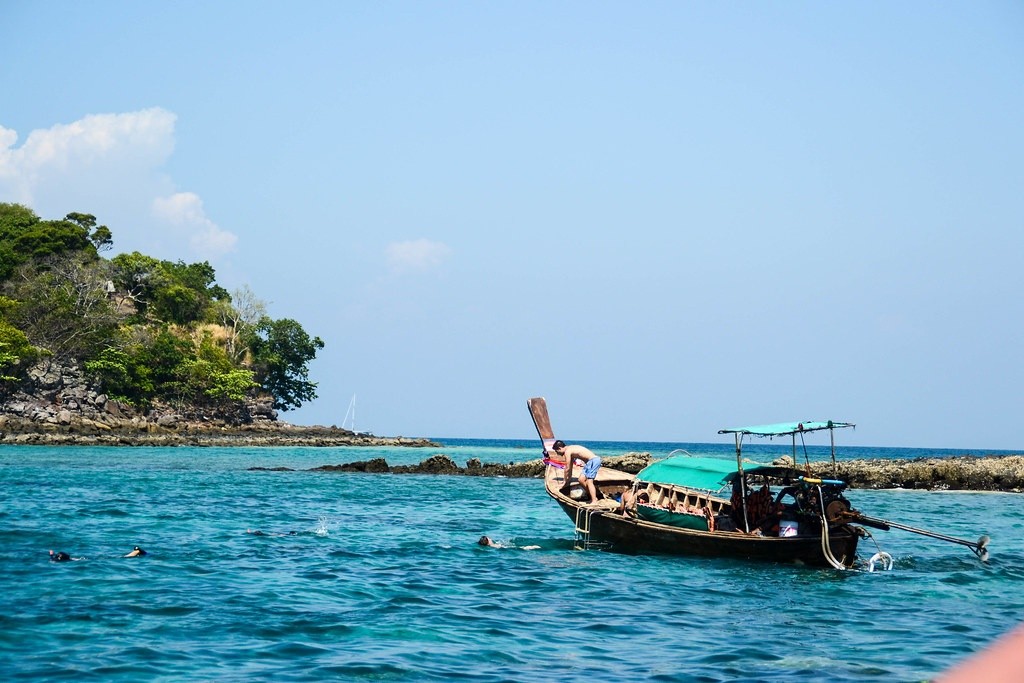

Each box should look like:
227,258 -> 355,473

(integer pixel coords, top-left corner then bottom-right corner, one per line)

526,395 -> 992,571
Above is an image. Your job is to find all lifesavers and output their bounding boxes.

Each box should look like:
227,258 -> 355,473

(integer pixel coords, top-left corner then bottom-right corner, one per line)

867,551 -> 894,573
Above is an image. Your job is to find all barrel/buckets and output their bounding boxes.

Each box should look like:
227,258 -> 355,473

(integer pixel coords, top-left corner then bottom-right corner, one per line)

779,521 -> 798,537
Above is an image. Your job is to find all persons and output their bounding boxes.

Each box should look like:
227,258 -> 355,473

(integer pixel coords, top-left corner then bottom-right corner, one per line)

809,496 -> 820,514
49,545 -> 148,562
479,536 -> 541,550
552,440 -> 602,505
